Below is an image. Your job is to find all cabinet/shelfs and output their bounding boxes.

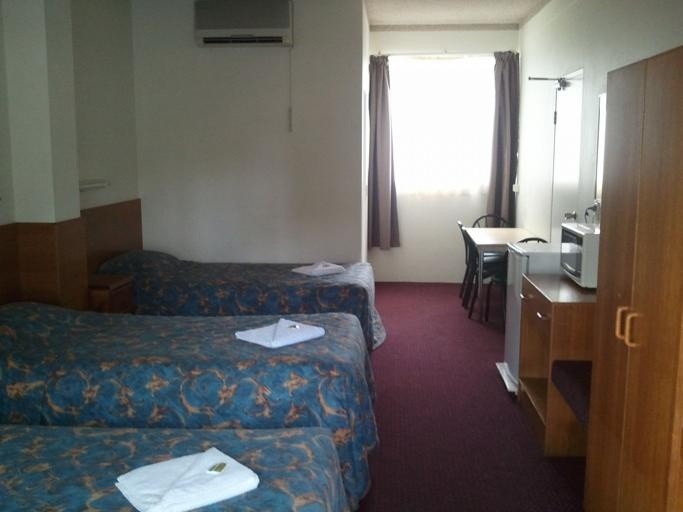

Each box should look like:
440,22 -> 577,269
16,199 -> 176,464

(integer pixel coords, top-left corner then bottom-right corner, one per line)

519,273 -> 597,458
579,44 -> 683,512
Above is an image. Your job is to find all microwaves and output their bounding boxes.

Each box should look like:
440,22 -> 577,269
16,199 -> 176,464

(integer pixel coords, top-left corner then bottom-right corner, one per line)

559,222 -> 599,292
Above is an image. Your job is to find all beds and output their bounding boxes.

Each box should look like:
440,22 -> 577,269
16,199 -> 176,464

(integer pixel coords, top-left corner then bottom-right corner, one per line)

1,427 -> 341,512
101,246 -> 378,336
1,301 -> 377,507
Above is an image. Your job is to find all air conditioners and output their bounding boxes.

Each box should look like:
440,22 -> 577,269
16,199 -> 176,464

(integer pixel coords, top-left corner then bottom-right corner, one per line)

193,0 -> 295,50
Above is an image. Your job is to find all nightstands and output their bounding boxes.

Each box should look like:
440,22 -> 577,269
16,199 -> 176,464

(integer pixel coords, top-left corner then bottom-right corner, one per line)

86,273 -> 138,314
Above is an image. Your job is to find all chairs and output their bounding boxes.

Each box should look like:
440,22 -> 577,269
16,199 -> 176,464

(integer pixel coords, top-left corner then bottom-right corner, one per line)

457,215 -> 549,323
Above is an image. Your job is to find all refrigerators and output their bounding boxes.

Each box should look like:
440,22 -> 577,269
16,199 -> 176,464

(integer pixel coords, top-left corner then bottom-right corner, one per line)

503,241 -> 560,386
